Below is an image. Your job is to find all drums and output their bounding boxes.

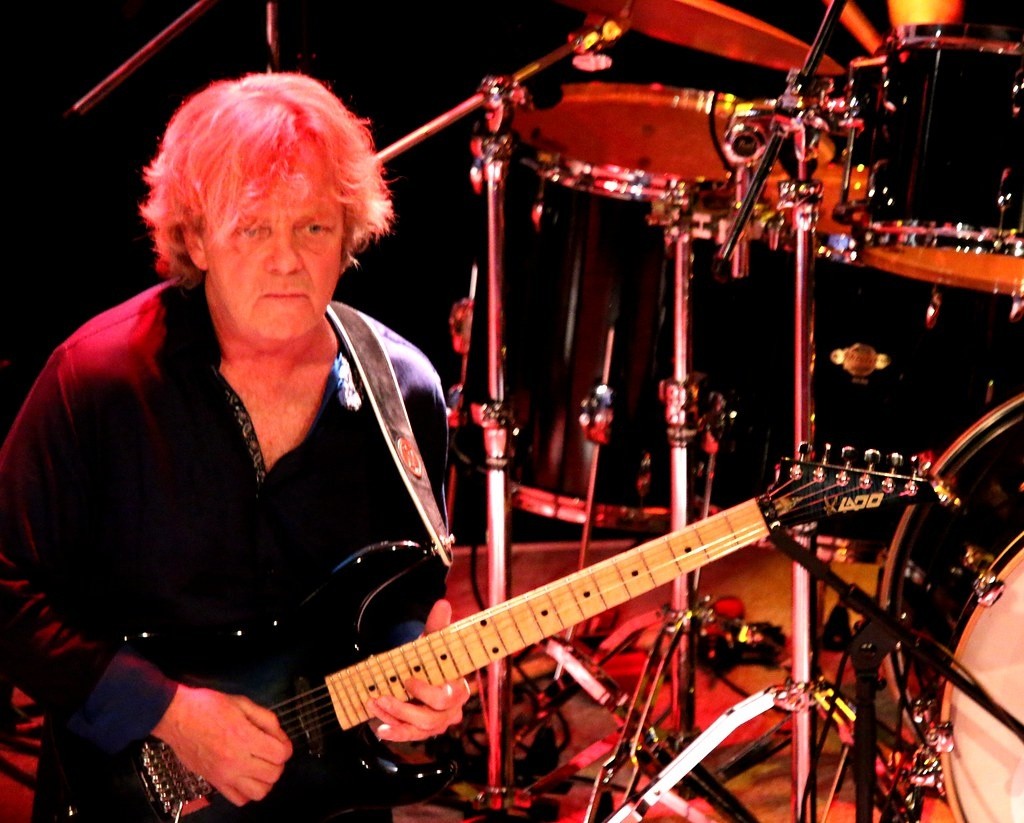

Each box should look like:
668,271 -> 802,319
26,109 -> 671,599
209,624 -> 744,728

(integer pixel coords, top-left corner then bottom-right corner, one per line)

818,246 -> 1024,464
451,81 -> 873,531
863,23 -> 1024,244
877,390 -> 1024,823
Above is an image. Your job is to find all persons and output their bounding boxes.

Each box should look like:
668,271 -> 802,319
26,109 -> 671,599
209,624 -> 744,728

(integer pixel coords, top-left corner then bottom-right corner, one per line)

0,73 -> 453,823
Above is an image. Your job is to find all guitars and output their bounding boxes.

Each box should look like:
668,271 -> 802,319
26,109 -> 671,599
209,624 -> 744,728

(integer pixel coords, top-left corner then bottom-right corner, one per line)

55,440 -> 954,823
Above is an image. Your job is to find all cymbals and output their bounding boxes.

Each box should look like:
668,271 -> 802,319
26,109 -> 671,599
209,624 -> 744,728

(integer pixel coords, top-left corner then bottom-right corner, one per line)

563,0 -> 846,78
